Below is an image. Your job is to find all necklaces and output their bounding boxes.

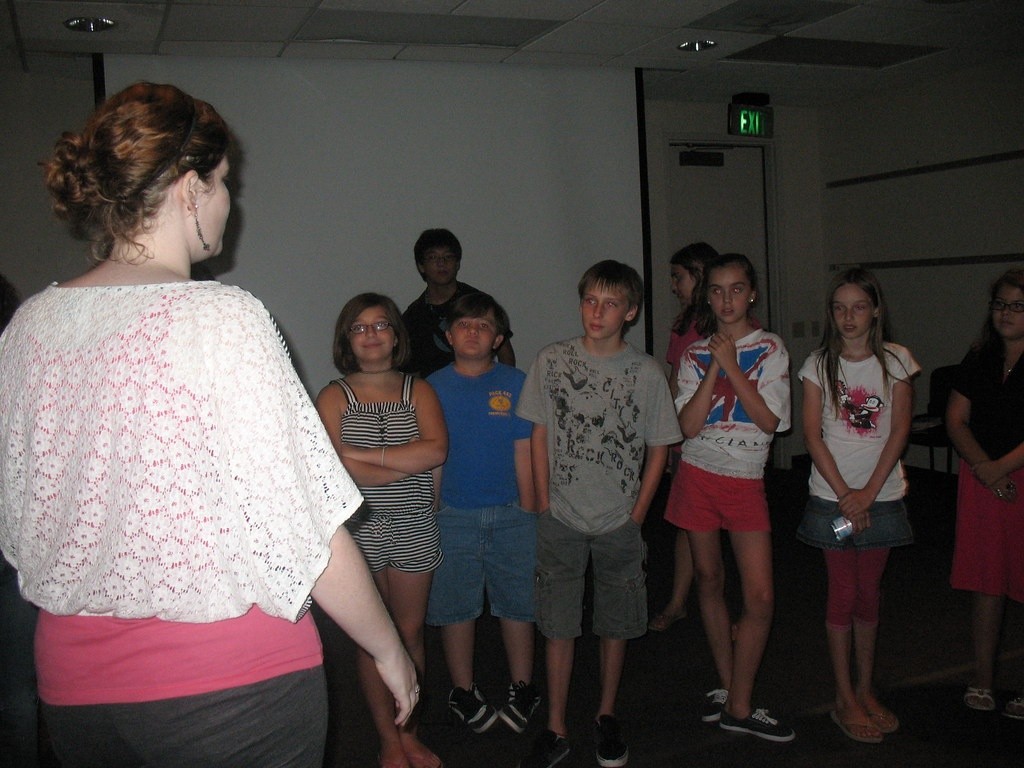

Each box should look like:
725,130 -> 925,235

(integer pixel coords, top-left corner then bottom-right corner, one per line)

360,366 -> 394,374
1004,368 -> 1011,375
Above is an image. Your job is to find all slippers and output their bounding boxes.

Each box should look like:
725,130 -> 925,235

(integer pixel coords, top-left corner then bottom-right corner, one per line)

831,709 -> 882,743
409,749 -> 443,768
377,750 -> 409,768
964,688 -> 993,710
1001,696 -> 1024,719
647,607 -> 687,630
866,709 -> 899,732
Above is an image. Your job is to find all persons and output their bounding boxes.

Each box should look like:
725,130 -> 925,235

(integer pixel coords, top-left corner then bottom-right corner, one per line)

425,292 -> 541,732
0,80 -> 421,768
399,229 -> 515,380
946,270 -> 1024,720
797,265 -> 922,742
664,253 -> 795,741
649,239 -> 761,634
312,293 -> 443,768
515,260 -> 683,768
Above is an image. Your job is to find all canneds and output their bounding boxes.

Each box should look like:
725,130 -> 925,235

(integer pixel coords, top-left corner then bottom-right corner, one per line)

827,516 -> 852,541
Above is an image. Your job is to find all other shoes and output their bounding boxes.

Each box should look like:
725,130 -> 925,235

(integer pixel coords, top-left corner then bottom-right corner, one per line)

595,714 -> 628,767
520,730 -> 569,768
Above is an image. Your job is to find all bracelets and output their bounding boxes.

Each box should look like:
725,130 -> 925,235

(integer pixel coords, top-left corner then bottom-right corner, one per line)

837,492 -> 851,499
381,446 -> 387,466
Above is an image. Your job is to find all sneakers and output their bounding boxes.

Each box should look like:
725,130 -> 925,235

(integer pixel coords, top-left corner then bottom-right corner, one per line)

499,680 -> 543,733
701,689 -> 729,722
720,704 -> 795,742
448,682 -> 498,733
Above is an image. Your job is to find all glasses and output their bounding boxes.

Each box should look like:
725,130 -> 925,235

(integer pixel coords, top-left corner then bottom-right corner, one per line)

350,320 -> 393,334
988,301 -> 1024,312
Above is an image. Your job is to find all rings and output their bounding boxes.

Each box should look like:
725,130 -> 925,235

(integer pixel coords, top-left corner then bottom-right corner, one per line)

995,481 -> 1016,496
416,685 -> 420,693
